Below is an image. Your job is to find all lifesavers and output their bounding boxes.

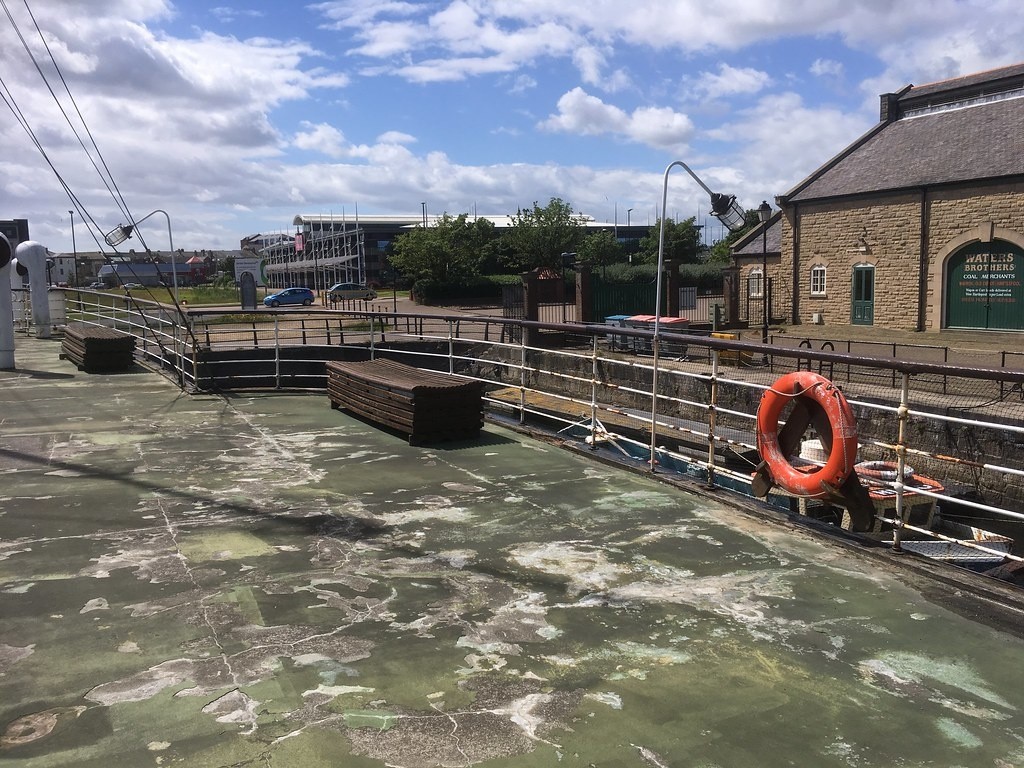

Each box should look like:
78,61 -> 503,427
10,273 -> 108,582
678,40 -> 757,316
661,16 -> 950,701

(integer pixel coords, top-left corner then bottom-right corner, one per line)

751,369 -> 856,496
852,457 -> 914,480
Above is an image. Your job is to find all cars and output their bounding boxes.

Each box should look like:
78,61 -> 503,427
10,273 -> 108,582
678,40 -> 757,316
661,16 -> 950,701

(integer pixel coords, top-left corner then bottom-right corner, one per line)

263,288 -> 315,307
326,283 -> 378,303
89,282 -> 111,289
58,281 -> 69,288
119,282 -> 144,290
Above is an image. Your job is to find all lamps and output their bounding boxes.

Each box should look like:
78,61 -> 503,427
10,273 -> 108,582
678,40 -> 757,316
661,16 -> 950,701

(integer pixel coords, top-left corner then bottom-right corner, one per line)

103,224 -> 133,248
705,193 -> 746,231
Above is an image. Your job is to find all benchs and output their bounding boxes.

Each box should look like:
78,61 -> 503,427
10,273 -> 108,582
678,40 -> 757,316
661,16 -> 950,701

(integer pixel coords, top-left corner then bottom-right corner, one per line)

325,358 -> 486,447
710,334 -> 754,366
57,325 -> 138,371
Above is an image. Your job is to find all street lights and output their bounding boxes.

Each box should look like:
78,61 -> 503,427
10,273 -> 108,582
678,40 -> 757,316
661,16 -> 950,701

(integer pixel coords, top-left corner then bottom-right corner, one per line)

68,210 -> 84,310
104,209 -> 182,370
651,157 -> 749,472
421,202 -> 425,233
627,208 -> 633,226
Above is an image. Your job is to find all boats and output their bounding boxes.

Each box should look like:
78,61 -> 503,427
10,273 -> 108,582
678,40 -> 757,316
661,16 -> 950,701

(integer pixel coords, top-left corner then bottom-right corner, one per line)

586,429 -> 1014,570
